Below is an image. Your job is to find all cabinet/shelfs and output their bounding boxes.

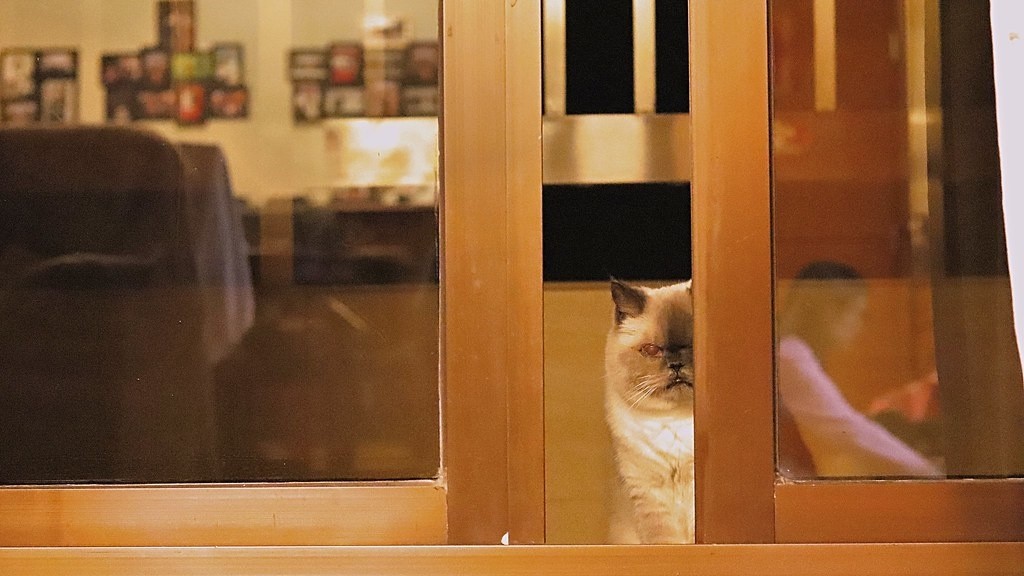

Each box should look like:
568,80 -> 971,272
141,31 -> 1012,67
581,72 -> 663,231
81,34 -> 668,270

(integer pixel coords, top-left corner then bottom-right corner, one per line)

240,202 -> 438,290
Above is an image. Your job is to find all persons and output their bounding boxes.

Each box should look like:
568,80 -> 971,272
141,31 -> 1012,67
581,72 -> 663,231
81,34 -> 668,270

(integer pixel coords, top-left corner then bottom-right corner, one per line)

779,260 -> 946,475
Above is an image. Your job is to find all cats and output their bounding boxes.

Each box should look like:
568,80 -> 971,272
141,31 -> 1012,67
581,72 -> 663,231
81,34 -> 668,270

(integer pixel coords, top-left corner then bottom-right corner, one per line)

603,274 -> 818,544
